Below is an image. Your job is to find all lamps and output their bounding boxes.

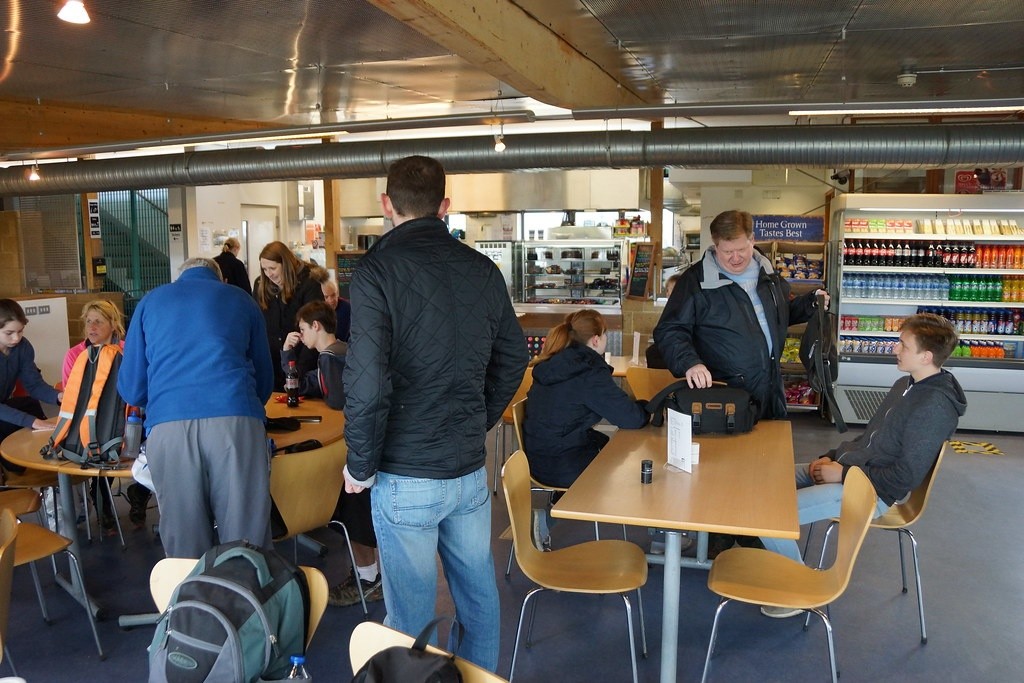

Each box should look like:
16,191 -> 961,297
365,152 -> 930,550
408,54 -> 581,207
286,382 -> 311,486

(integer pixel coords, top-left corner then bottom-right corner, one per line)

493,123 -> 506,152
30,159 -> 40,180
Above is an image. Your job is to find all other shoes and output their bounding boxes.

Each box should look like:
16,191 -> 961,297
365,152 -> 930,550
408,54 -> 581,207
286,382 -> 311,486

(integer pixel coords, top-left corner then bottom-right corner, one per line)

271,515 -> 289,542
760,605 -> 805,618
127,482 -> 152,525
89,488 -> 118,536
704,532 -> 736,559
531,508 -> 552,552
651,536 -> 693,554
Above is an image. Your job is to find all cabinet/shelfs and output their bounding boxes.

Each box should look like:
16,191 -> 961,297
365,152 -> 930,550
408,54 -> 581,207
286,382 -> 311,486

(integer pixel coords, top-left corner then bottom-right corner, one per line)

752,240 -> 827,410
522,242 -> 630,305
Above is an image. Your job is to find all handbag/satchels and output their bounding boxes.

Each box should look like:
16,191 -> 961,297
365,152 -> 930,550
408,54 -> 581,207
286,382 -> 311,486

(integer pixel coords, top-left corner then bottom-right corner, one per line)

645,379 -> 755,435
798,295 -> 849,434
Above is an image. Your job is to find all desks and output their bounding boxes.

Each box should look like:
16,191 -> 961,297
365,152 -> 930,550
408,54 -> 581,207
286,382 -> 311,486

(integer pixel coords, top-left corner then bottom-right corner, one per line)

0,391 -> 348,621
550,420 -> 800,683
603,355 -> 648,377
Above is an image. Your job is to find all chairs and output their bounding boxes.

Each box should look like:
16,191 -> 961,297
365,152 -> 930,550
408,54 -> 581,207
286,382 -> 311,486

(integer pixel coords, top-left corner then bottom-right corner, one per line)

507,399 -> 629,578
492,369 -> 534,495
802,440 -> 947,644
150,559 -> 329,683
625,368 -> 679,402
269,437 -> 367,615
0,470 -> 124,683
700,467 -> 878,683
350,621 -> 508,683
501,449 -> 648,683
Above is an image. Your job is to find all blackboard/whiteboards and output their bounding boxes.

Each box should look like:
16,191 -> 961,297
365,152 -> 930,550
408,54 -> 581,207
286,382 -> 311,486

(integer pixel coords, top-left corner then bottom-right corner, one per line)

626,241 -> 655,300
334,250 -> 367,300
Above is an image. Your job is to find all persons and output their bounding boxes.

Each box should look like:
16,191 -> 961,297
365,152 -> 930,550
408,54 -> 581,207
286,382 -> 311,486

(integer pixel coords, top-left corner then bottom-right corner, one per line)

652,211 -> 830,418
251,246 -> 329,392
62,300 -> 152,536
280,299 -> 349,410
0,299 -> 64,476
115,260 -> 277,560
519,310 -> 692,554
328,482 -> 382,605
213,240 -> 253,295
751,313 -> 968,619
342,155 -> 529,673
320,278 -> 349,342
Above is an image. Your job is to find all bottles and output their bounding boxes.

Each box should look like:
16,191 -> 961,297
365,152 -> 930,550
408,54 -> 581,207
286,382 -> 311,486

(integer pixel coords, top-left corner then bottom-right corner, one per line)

123,411 -> 142,458
286,361 -> 299,407
285,655 -> 308,681
840,240 -> 1024,360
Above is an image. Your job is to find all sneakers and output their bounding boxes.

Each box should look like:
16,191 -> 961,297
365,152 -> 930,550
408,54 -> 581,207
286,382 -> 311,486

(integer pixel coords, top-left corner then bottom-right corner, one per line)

328,564 -> 384,606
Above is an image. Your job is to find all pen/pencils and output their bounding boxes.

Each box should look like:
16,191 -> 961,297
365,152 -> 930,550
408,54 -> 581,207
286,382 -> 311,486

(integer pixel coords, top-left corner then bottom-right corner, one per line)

32,429 -> 55,432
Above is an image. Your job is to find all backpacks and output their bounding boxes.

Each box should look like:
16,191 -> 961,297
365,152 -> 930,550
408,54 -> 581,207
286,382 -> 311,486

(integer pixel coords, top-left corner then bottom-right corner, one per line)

147,538 -> 312,683
349,618 -> 464,683
38,343 -> 130,471
